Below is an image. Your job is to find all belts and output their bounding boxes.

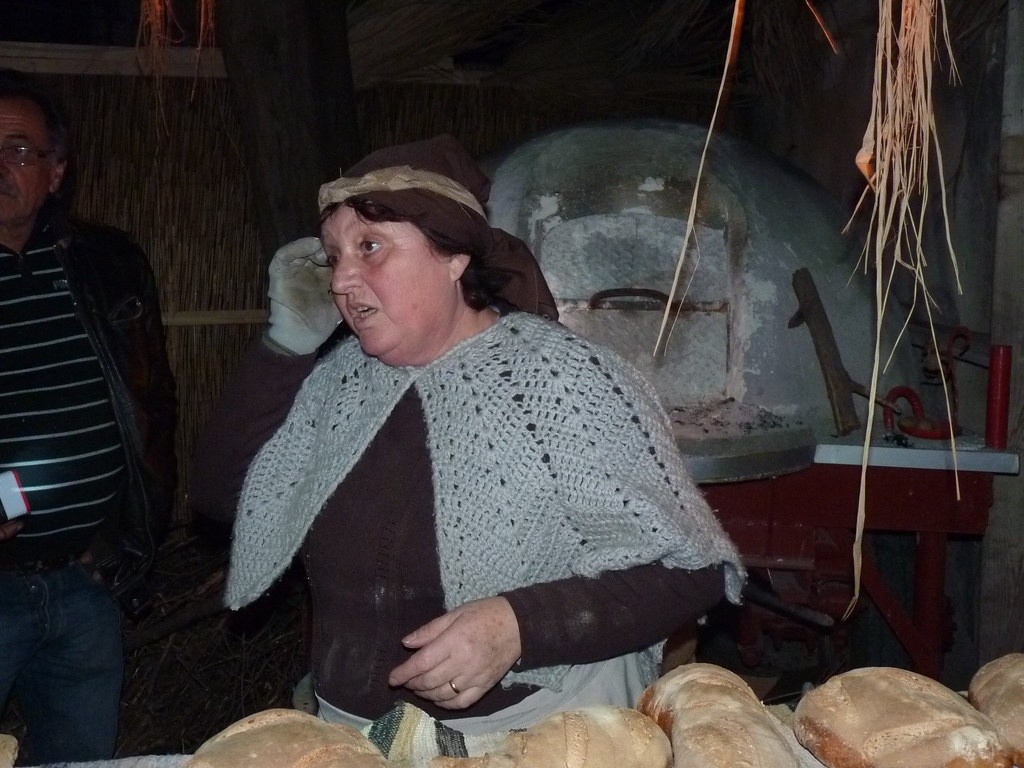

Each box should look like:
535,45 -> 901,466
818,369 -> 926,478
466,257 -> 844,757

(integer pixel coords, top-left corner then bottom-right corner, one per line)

0,550 -> 82,572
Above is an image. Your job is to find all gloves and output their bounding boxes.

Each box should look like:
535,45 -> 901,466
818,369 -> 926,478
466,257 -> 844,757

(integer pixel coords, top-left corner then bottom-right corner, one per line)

268,236 -> 342,355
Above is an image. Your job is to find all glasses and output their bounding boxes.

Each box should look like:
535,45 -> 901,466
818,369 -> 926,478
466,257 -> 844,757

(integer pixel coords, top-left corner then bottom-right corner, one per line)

0,144 -> 55,166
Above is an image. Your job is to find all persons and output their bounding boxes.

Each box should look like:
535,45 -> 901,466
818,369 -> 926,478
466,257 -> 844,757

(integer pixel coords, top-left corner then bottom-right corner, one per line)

0,70 -> 179,763
190,134 -> 749,756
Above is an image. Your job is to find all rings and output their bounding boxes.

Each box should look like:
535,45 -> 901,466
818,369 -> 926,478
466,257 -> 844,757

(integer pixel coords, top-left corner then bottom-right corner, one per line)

449,680 -> 461,693
